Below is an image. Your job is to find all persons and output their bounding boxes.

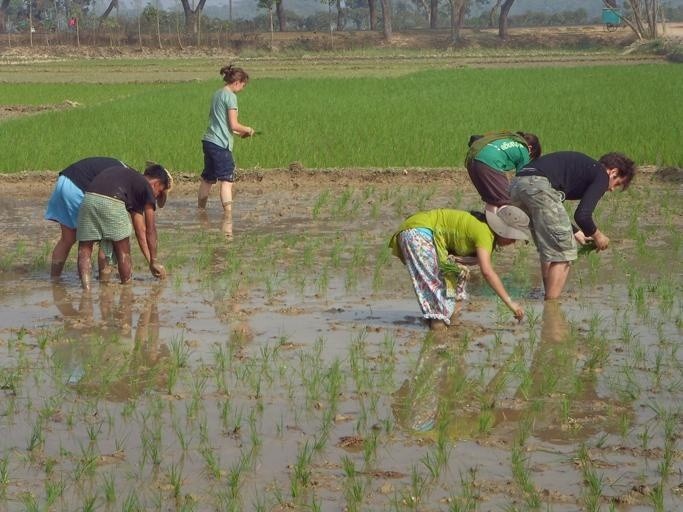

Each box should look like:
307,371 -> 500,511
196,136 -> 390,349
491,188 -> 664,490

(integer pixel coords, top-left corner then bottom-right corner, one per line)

196,210 -> 256,346
196,64 -> 254,211
392,329 -> 525,442
466,244 -> 542,298
507,300 -> 638,446
49,281 -> 175,404
387,204 -> 531,333
463,130 -> 542,213
75,163 -> 170,291
508,151 -> 637,300
44,155 -> 173,281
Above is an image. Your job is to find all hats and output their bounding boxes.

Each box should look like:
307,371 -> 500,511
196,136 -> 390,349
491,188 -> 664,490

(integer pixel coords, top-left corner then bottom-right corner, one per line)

484,204 -> 530,245
145,161 -> 173,207
